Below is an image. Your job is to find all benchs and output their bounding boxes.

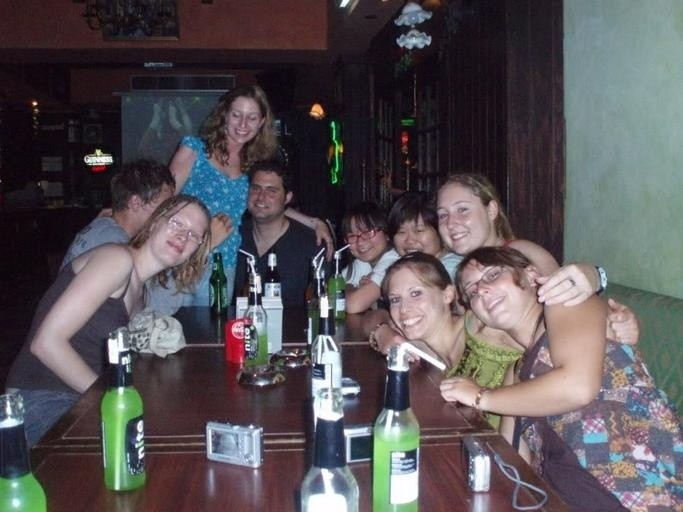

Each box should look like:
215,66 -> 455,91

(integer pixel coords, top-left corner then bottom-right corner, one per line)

601,282 -> 683,421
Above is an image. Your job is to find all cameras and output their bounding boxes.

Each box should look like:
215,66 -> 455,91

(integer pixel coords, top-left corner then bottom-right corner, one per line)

343,378 -> 361,399
205,421 -> 263,469
342,423 -> 372,466
460,435 -> 491,493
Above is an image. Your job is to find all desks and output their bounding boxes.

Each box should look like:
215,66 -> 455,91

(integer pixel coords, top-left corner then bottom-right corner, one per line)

0,204 -> 109,282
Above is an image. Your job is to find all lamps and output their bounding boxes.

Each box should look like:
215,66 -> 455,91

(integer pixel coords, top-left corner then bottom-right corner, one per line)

336,0 -> 359,15
393,0 -> 434,50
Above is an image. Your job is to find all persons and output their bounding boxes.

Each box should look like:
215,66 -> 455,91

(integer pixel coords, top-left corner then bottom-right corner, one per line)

326,167 -> 682,511
94,83 -> 336,344
1,157 -> 331,448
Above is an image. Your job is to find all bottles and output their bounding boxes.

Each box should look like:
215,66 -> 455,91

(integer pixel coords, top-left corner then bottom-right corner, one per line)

97,324 -> 149,492
204,244 -> 348,377
0,393 -> 48,511
300,344 -> 421,511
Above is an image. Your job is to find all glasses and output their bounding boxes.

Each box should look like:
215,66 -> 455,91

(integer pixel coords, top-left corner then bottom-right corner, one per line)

464,264 -> 508,302
161,214 -> 207,246
339,228 -> 383,242
